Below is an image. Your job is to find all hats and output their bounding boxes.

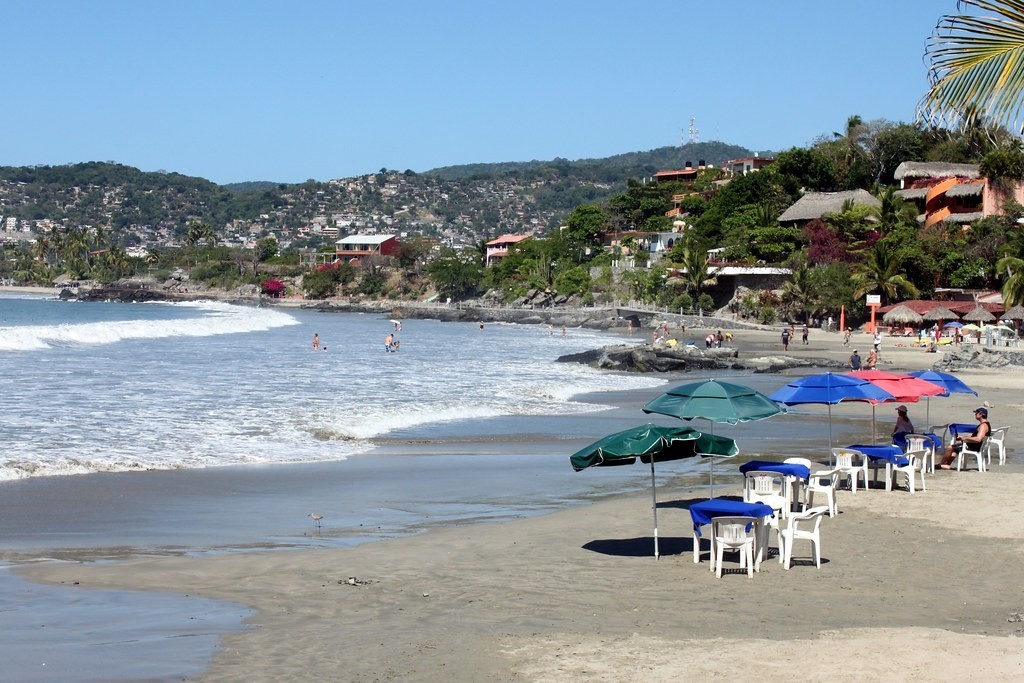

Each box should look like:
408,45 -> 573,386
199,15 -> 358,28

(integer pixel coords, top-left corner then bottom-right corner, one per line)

895,405 -> 908,413
803,324 -> 806,326
973,408 -> 988,416
853,349 -> 857,352
848,327 -> 852,330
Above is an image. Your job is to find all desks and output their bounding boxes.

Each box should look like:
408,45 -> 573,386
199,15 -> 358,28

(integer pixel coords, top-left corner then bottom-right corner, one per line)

949,423 -> 977,444
896,433 -> 942,473
846,445 -> 909,490
739,460 -> 810,512
689,499 -> 774,572
998,337 -> 1017,345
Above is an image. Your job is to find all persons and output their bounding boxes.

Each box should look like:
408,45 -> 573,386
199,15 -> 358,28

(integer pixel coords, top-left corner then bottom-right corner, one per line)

934,408 -> 991,470
742,309 -> 882,371
314,322 -> 401,354
547,324 -> 567,336
480,321 -> 484,330
628,319 -> 734,347
892,405 -> 914,437
892,323 -> 995,351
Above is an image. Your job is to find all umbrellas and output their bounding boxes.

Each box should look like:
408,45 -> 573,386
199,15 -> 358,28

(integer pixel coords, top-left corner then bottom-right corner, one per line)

769,372 -> 896,485
569,423 -> 740,561
905,369 -> 978,433
642,378 -> 787,499
922,306 -> 960,329
999,305 -> 1024,329
883,305 -> 922,327
962,306 -> 996,327
840,369 -> 945,446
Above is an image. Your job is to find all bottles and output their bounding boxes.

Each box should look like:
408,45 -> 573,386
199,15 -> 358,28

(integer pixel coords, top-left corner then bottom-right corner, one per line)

955,432 -> 958,441
837,440 -> 839,448
930,428 -> 933,434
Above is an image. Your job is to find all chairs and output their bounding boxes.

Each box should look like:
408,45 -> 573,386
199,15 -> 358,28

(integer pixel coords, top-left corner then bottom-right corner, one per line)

711,423 -> 1010,579
